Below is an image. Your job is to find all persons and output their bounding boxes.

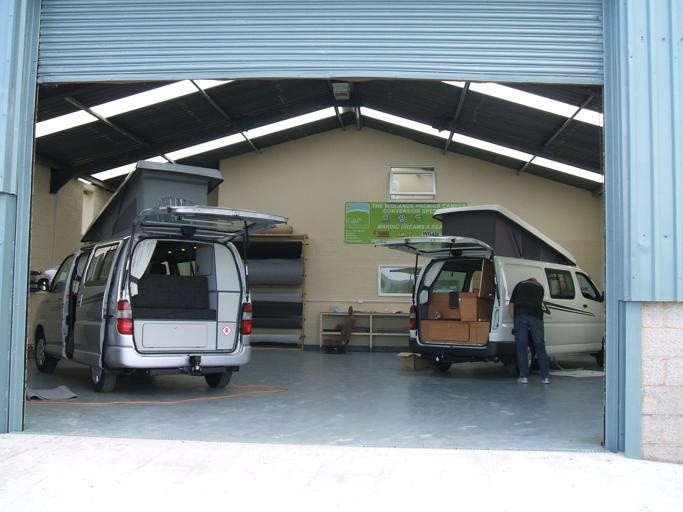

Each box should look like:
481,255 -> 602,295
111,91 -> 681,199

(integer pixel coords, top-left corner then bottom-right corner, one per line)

508,277 -> 550,384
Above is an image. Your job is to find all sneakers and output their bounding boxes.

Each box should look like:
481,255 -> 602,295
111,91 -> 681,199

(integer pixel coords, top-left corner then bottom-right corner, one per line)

540,377 -> 551,384
517,377 -> 528,384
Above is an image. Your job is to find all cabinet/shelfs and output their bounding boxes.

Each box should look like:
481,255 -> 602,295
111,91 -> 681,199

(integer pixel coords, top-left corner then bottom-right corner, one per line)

239,233 -> 308,352
318,311 -> 408,351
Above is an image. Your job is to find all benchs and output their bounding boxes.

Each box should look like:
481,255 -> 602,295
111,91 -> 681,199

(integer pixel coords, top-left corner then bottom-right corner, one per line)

132,276 -> 216,319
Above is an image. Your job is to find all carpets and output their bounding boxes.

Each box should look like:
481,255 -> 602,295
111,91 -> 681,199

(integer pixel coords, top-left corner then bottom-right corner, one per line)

534,369 -> 605,377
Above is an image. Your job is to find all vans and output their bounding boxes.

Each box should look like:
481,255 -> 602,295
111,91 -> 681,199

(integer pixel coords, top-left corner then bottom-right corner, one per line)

371,203 -> 604,379
32,159 -> 289,392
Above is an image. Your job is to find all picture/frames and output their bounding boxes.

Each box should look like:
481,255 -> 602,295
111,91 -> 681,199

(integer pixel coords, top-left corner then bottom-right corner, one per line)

378,264 -> 425,297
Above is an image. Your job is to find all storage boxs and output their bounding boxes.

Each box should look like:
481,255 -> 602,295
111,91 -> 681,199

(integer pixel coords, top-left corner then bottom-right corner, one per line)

396,352 -> 431,369
419,291 -> 490,344
